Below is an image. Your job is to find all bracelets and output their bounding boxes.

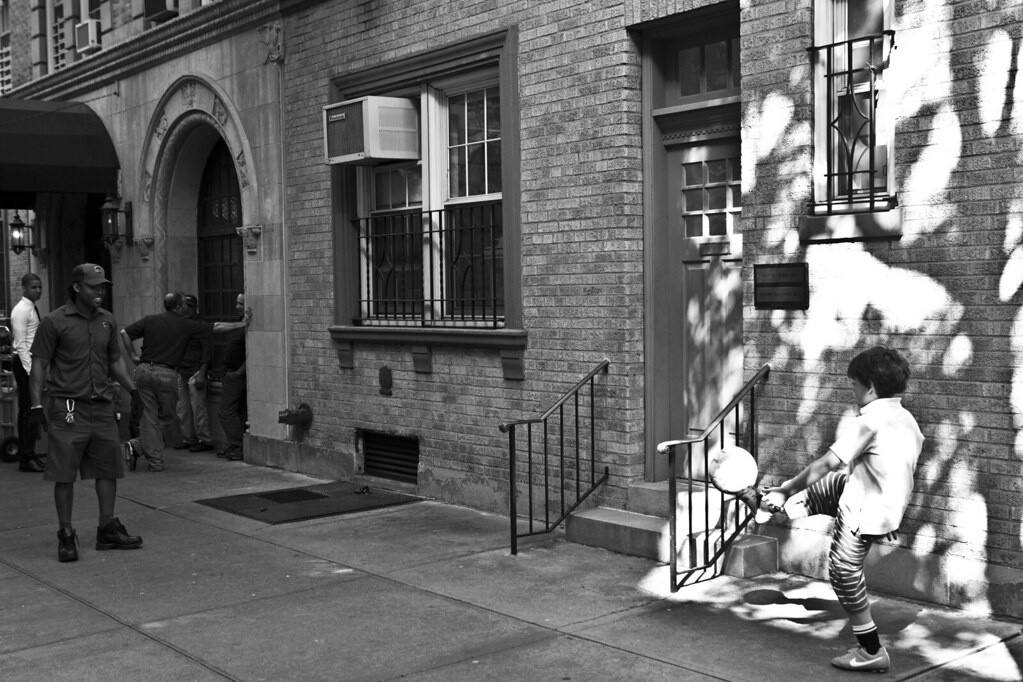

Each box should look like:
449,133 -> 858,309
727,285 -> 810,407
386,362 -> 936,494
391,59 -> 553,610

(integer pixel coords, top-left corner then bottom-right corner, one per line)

30,405 -> 42,410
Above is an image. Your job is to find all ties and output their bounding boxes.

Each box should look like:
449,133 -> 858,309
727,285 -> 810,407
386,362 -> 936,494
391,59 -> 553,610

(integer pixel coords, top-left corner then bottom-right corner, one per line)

34,305 -> 41,322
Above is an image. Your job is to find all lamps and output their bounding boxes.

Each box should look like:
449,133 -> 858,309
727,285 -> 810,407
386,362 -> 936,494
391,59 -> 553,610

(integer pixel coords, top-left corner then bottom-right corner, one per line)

10,210 -> 39,258
99,191 -> 133,247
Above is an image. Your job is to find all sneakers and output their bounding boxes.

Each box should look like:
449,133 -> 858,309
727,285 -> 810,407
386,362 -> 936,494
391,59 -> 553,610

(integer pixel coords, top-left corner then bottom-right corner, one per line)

830,642 -> 891,673
736,486 -> 786,526
57,526 -> 81,562
96,518 -> 143,551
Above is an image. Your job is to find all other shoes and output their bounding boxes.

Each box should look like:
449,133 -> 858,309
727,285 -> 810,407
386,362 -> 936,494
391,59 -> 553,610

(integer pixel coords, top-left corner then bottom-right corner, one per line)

173,440 -> 215,452
125,442 -> 139,472
18,456 -> 48,472
216,444 -> 243,460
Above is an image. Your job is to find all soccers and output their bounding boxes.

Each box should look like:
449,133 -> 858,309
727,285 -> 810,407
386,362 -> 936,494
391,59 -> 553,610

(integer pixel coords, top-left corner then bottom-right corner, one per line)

708,445 -> 759,494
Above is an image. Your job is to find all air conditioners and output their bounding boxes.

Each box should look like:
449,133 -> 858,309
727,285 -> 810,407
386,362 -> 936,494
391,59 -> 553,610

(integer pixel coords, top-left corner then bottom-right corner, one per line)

144,0 -> 179,23
75,19 -> 102,55
322,95 -> 421,166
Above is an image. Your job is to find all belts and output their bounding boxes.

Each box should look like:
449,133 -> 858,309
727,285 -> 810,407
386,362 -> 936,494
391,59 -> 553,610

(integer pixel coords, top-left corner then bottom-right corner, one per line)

140,360 -> 180,373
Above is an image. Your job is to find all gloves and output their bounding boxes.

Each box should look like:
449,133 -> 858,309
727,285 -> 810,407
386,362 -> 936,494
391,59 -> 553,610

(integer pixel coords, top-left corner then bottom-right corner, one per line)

29,404 -> 49,441
129,389 -> 144,421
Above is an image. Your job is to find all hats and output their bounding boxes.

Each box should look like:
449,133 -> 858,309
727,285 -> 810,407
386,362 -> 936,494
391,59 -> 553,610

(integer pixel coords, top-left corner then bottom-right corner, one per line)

184,294 -> 199,307
72,262 -> 114,287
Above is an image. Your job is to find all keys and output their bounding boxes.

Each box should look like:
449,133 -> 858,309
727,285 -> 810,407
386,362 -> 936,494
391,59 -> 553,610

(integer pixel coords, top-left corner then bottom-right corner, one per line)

65,413 -> 74,422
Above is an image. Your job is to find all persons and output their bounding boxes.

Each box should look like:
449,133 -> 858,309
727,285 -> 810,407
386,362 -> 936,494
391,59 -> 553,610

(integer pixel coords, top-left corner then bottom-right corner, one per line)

30,263 -> 146,563
736,346 -> 925,673
119,264 -> 252,472
11,273 -> 43,472
